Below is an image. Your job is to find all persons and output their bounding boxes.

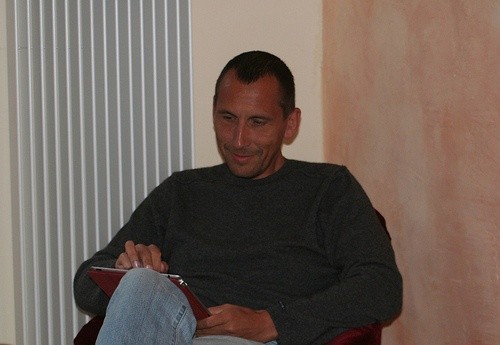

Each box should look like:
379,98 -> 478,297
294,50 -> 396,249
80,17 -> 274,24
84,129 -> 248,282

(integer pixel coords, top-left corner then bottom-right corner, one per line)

73,50 -> 403,345
